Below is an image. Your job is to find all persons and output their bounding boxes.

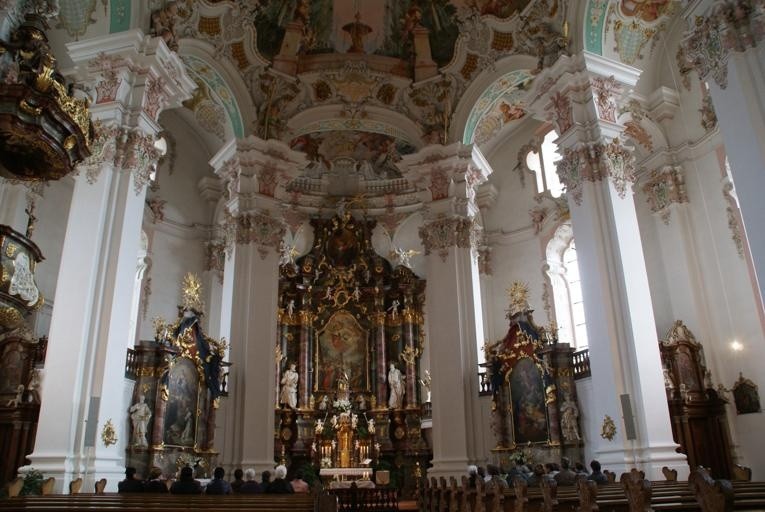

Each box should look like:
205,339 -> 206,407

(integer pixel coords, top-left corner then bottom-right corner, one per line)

280,364 -> 299,410
115,465 -> 310,493
388,361 -> 405,410
460,456 -> 607,486
129,396 -> 152,450
418,369 -> 430,401
